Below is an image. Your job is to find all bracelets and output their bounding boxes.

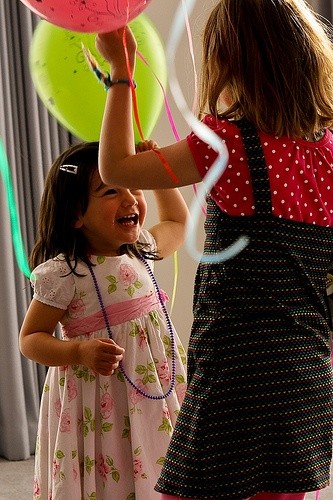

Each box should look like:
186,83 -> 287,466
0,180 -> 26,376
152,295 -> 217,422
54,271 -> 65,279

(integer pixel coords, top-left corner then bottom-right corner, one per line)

80,41 -> 138,93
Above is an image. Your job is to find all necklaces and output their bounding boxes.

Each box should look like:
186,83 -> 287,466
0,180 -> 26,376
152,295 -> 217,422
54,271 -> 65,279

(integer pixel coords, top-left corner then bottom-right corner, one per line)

82,245 -> 174,400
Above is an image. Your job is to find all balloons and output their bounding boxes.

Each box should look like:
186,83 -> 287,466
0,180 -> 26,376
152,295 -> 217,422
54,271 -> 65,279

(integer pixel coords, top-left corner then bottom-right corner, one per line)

27,12 -> 166,143
18,1 -> 154,33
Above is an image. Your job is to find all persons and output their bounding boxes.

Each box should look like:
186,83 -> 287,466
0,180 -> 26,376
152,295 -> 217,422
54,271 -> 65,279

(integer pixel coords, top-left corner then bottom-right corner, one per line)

17,140 -> 193,500
80,0 -> 333,500
